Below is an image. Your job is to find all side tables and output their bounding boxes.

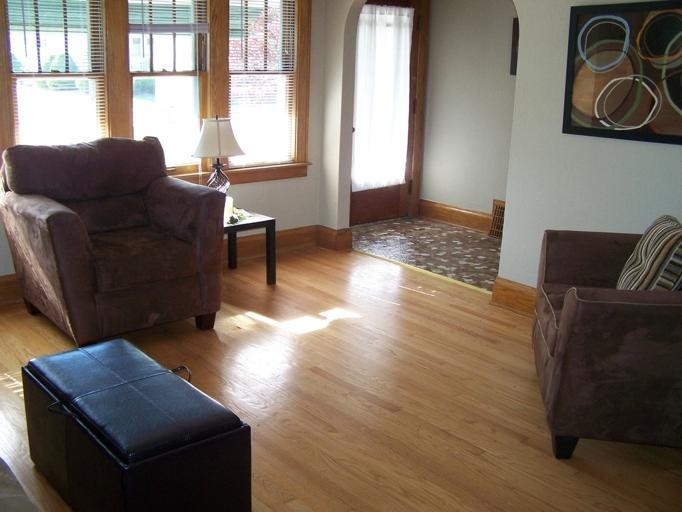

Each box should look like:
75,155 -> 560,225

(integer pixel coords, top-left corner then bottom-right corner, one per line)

223,208 -> 276,285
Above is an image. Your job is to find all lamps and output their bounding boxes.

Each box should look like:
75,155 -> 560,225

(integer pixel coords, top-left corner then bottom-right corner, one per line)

190,117 -> 245,193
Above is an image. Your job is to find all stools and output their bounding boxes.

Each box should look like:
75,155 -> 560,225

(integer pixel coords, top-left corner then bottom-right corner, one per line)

21,337 -> 251,510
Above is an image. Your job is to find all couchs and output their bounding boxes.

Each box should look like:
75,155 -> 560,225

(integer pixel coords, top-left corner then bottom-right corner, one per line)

1,135 -> 226,349
535,212 -> 682,461
